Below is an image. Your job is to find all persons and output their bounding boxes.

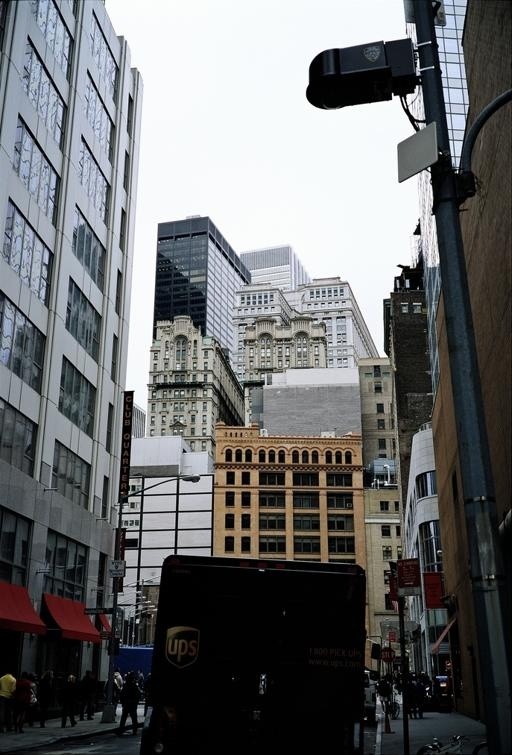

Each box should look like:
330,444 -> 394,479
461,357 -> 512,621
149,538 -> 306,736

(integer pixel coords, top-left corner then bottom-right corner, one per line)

378,669 -> 453,719
0,668 -> 151,736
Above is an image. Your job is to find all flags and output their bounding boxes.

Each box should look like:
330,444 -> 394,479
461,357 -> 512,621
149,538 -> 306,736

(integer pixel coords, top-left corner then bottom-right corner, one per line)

389,589 -> 398,612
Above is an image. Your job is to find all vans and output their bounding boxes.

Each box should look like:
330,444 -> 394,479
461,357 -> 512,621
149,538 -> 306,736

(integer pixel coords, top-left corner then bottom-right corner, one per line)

141,552 -> 367,749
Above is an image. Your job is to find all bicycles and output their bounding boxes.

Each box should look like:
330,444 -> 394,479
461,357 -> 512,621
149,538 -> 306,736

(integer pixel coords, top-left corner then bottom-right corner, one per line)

416,733 -> 486,754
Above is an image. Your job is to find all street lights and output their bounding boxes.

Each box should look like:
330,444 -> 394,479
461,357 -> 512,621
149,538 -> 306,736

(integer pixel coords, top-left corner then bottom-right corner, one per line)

99,474 -> 200,722
119,587 -> 156,645
